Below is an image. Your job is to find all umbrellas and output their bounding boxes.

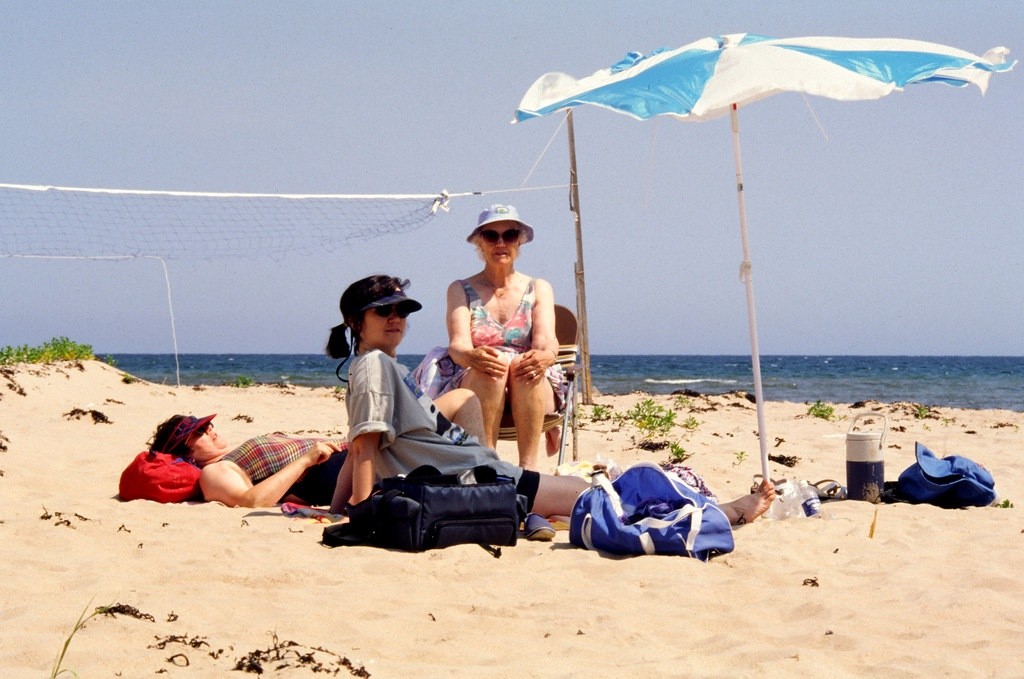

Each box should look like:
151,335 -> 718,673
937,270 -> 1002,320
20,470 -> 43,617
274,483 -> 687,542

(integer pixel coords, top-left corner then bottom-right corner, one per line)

510,29 -> 1017,487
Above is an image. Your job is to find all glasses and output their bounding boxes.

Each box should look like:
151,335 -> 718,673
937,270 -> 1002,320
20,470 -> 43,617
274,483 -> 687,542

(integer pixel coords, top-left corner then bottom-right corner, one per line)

479,229 -> 522,243
374,306 -> 411,318
188,421 -> 213,446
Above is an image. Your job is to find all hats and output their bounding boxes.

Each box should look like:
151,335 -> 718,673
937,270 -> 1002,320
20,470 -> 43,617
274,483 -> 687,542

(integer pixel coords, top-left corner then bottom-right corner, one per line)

339,274 -> 422,322
163,413 -> 217,453
466,203 -> 534,247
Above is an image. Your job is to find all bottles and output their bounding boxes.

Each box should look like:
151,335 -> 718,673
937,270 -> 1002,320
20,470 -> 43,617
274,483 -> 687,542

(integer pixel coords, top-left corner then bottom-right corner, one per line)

796,479 -> 823,520
845,411 -> 886,498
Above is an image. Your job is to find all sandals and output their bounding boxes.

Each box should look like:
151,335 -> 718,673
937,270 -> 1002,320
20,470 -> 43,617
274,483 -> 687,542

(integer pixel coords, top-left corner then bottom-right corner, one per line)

808,479 -> 847,502
749,474 -> 787,495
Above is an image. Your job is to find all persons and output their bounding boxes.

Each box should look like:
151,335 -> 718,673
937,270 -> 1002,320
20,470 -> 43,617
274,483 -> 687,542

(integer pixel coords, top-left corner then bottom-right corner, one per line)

445,205 -> 560,541
146,389 -> 487,507
325,274 -> 775,527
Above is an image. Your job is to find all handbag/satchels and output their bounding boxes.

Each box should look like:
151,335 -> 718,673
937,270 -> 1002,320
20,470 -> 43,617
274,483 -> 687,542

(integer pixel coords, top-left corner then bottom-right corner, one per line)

570,463 -> 734,564
898,441 -> 996,507
321,464 -> 520,559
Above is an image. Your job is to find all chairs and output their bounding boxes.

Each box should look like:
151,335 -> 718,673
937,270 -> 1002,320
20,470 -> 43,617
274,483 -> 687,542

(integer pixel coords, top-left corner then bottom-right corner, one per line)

413,305 -> 580,475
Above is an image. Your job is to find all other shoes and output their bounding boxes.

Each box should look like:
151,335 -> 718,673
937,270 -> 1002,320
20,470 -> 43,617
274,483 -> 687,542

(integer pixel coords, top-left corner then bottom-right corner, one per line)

524,515 -> 556,541
